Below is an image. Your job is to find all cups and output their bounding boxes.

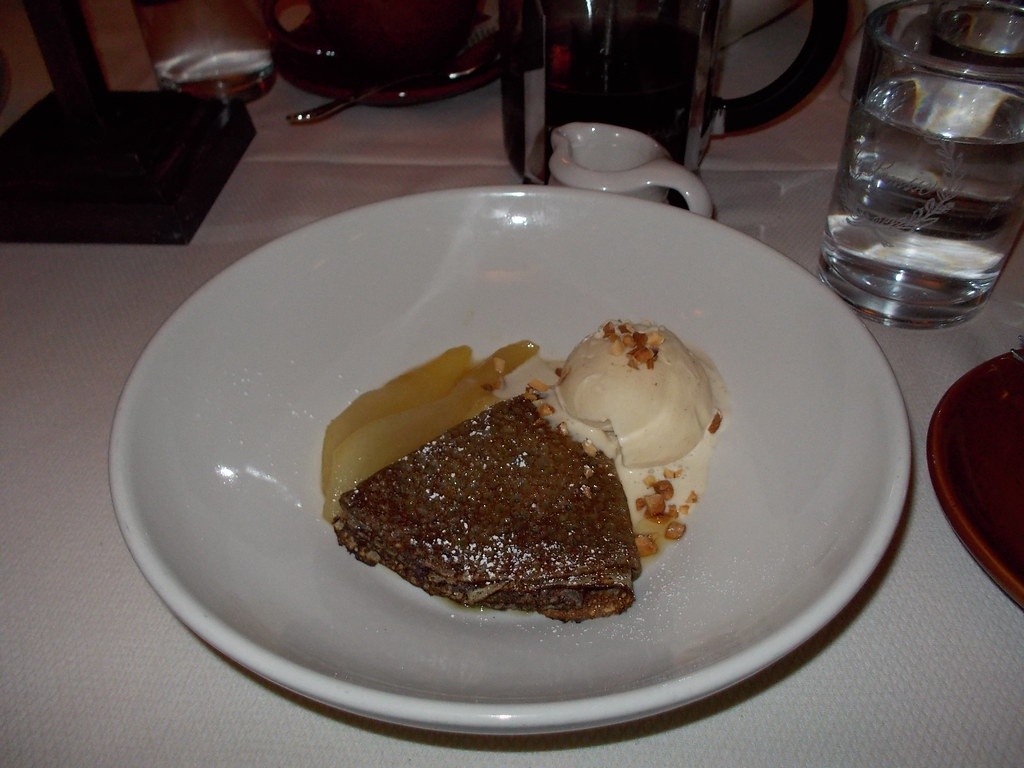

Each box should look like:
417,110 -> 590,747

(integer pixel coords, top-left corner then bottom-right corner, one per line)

269,0 -> 487,67
495,0 -> 866,186
131,0 -> 275,107
549,121 -> 714,218
820,0 -> 1024,328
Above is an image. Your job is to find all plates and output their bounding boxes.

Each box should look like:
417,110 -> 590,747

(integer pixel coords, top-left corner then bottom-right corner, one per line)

927,343 -> 1024,614
110,189 -> 911,733
266,20 -> 500,105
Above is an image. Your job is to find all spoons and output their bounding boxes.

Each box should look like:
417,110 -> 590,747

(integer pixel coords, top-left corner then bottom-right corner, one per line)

287,32 -> 502,128
